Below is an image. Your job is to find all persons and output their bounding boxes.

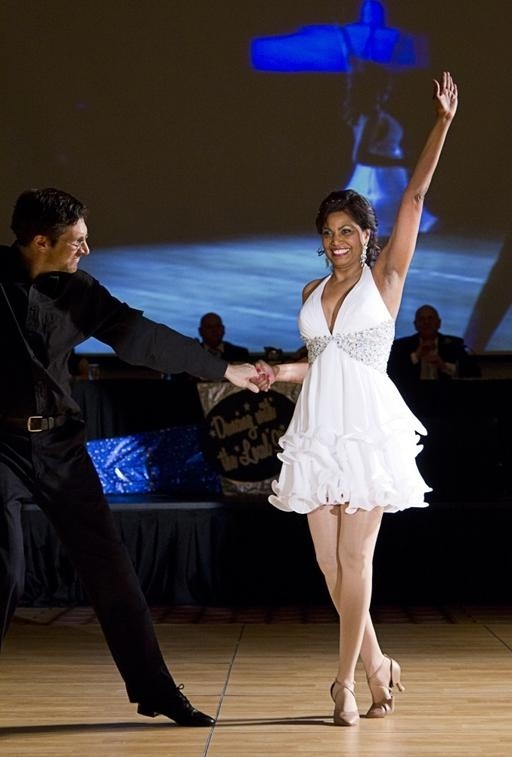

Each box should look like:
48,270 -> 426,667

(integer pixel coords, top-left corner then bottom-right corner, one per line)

388,304 -> 480,380
198,312 -> 251,367
251,66 -> 460,728
326,55 -> 439,257
0,184 -> 261,727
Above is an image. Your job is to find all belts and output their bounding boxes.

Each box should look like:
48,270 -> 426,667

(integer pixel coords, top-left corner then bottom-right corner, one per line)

6,414 -> 71,433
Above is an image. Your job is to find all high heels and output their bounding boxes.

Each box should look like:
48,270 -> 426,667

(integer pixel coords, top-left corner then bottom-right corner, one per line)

365,657 -> 403,718
329,678 -> 361,727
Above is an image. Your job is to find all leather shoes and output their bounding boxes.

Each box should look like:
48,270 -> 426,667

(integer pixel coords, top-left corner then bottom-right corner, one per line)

135,686 -> 216,727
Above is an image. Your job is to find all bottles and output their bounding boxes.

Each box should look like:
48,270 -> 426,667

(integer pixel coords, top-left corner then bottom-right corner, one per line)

79,355 -> 89,380
88,363 -> 101,381
